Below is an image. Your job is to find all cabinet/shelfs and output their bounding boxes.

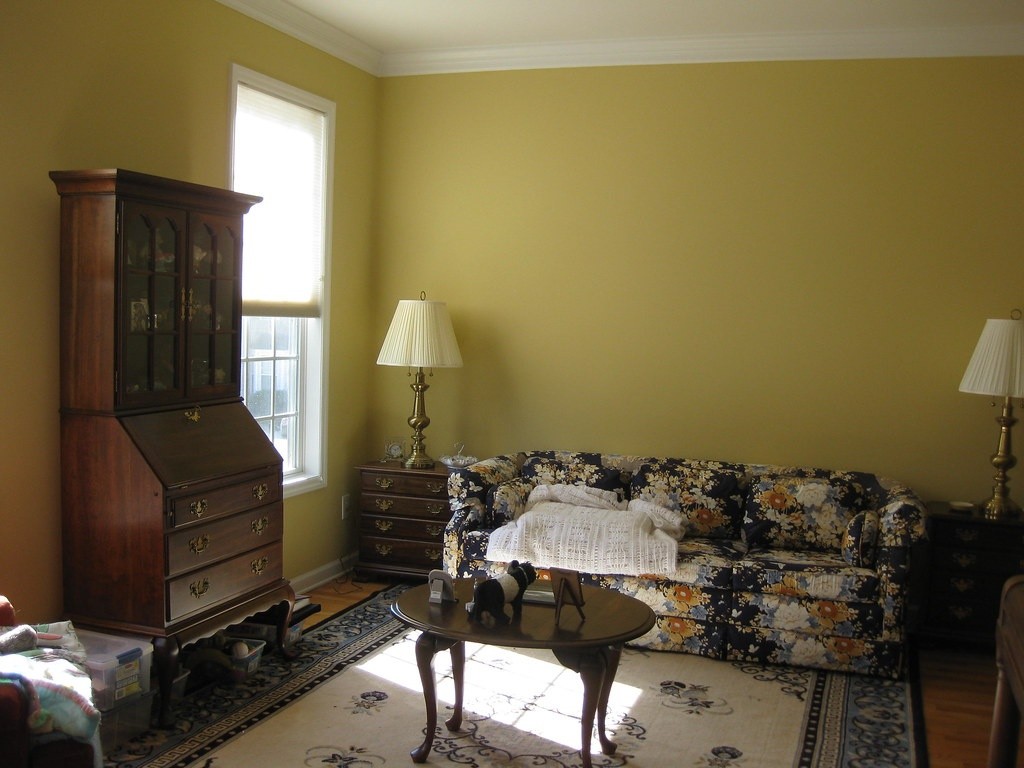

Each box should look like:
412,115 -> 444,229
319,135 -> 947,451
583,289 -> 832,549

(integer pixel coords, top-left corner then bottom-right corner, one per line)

49,168 -> 297,731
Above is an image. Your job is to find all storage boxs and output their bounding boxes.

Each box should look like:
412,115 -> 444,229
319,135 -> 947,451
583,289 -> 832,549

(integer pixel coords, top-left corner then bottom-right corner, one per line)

230,620 -> 302,652
199,636 -> 266,681
100,690 -> 152,752
152,667 -> 191,719
75,627 -> 154,712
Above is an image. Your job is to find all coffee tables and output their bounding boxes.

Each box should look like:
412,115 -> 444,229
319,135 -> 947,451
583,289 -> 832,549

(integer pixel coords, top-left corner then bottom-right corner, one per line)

390,577 -> 657,768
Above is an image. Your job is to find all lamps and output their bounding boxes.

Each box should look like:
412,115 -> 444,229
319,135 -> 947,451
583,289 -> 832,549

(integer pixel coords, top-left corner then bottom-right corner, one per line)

377,290 -> 465,469
959,307 -> 1024,519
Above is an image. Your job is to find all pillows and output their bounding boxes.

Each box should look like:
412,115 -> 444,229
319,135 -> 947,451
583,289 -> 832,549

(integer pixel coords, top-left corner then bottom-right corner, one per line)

741,472 -> 865,553
487,477 -> 535,531
521,457 -> 621,504
629,464 -> 743,541
841,510 -> 879,567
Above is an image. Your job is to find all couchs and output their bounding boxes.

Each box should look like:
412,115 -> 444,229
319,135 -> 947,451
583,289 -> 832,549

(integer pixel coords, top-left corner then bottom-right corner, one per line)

0,595 -> 102,768
443,451 -> 926,681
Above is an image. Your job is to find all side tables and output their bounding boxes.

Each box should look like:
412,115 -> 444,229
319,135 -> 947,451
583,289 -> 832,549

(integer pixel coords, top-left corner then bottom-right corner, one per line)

913,499 -> 1024,654
352,460 -> 455,582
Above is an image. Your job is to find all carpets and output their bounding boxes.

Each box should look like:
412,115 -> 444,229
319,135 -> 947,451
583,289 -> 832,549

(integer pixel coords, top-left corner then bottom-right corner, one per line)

102,589 -> 931,768
